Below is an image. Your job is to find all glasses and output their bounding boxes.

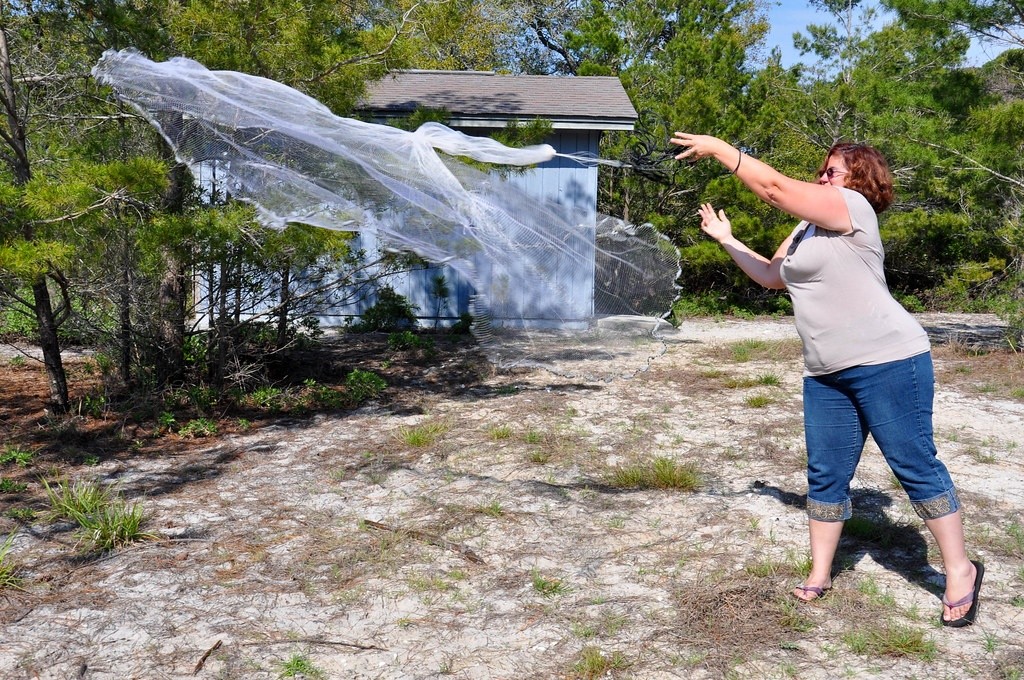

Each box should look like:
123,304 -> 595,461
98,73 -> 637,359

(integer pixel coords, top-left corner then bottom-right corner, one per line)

819,169 -> 848,176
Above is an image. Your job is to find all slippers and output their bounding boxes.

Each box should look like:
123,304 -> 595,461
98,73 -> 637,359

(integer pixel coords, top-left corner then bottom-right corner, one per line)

940,560 -> 985,628
792,583 -> 833,602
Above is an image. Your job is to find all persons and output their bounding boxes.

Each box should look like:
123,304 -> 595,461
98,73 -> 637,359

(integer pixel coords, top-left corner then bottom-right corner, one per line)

671,131 -> 984,627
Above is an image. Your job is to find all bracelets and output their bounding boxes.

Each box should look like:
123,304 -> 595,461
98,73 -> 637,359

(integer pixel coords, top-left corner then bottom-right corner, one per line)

718,147 -> 741,179
